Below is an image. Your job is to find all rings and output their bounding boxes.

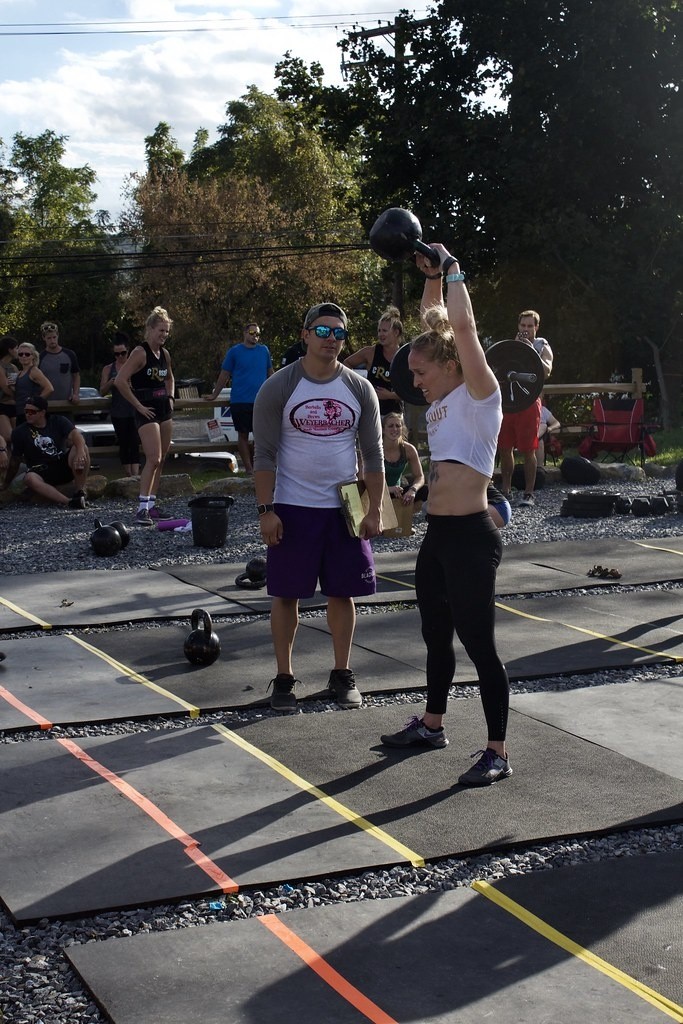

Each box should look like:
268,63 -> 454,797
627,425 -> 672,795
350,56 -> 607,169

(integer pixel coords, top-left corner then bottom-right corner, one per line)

81,458 -> 86,461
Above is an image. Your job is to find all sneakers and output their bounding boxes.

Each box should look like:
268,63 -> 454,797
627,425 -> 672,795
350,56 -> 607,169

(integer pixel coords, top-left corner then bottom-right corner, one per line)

265,672 -> 301,711
134,508 -> 153,524
326,668 -> 363,707
148,505 -> 174,521
458,747 -> 514,784
380,715 -> 449,749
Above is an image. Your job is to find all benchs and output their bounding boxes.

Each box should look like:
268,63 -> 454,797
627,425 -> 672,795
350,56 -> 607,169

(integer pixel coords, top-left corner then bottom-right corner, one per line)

86,440 -> 253,456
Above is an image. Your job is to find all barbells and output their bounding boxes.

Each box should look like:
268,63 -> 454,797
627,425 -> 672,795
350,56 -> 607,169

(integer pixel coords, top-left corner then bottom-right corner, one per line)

388,339 -> 546,413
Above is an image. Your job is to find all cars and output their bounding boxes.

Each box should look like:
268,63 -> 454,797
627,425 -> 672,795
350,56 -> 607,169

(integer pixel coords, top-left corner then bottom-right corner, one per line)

174,377 -> 215,398
74,387 -> 109,420
353,363 -> 368,378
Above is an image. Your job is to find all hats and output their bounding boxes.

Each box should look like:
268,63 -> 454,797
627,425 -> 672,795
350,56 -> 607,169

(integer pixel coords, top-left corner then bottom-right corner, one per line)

301,303 -> 348,352
26,396 -> 49,411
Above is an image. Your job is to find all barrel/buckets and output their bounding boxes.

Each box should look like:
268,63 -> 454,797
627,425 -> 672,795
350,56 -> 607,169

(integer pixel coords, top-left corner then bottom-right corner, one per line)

188,495 -> 235,548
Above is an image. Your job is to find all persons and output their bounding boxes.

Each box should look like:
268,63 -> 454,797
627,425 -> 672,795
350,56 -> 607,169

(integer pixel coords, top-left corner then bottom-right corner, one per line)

201,241 -> 559,526
0,305 -> 175,525
380,242 -> 513,787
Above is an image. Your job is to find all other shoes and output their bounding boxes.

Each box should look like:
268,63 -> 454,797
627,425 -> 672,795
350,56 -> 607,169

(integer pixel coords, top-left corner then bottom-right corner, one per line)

505,492 -> 514,501
68,495 -> 85,509
519,492 -> 536,506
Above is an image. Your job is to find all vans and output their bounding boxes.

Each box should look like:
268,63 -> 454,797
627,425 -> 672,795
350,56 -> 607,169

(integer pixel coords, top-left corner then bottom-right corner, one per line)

213,389 -> 254,442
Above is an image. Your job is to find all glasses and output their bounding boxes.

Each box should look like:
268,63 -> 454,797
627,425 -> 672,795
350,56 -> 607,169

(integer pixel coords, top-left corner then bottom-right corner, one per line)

306,324 -> 350,341
114,349 -> 128,357
42,325 -> 59,331
24,408 -> 42,415
244,330 -> 261,336
18,352 -> 33,357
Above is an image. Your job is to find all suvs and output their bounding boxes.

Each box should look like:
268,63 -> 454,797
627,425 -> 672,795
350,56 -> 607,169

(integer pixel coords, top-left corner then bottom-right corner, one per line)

75,423 -> 240,474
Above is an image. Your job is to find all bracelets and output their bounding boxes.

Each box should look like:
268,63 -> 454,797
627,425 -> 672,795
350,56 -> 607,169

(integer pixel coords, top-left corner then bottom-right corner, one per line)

410,487 -> 415,491
444,270 -> 467,285
255,503 -> 273,514
424,271 -> 442,280
168,395 -> 175,401
441,256 -> 459,276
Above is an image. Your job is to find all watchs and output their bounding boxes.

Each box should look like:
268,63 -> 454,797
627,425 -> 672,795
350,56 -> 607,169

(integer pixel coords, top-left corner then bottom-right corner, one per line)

252,302 -> 385,711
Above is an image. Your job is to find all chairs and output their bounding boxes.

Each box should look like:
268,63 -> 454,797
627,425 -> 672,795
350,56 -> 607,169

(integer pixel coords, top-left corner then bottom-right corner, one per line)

579,394 -> 660,467
497,398 -> 564,469
